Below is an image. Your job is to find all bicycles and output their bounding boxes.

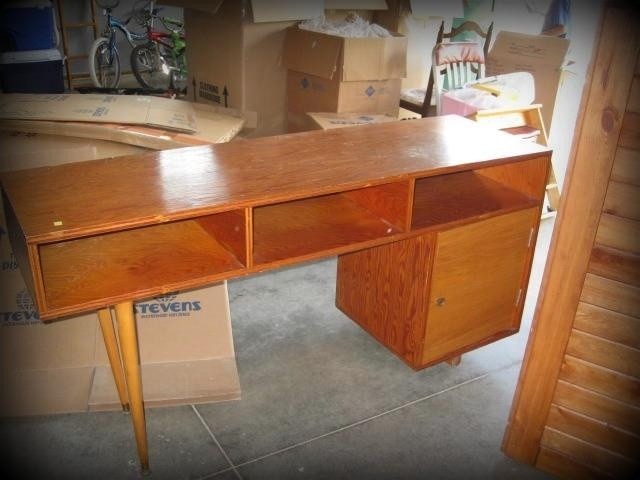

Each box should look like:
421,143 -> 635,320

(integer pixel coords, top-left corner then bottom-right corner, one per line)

145,15 -> 187,93
125,7 -> 187,92
88,0 -> 162,90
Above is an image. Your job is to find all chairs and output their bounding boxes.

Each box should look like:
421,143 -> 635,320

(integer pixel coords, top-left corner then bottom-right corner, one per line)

429,41 -> 485,120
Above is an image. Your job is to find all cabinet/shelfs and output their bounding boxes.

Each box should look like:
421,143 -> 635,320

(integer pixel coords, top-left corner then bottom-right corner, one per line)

473,104 -> 563,223
28,153 -> 553,371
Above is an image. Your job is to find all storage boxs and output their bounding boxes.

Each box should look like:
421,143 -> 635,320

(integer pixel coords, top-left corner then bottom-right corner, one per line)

487,30 -> 571,142
153,0 -> 408,130
1,88 -> 242,420
